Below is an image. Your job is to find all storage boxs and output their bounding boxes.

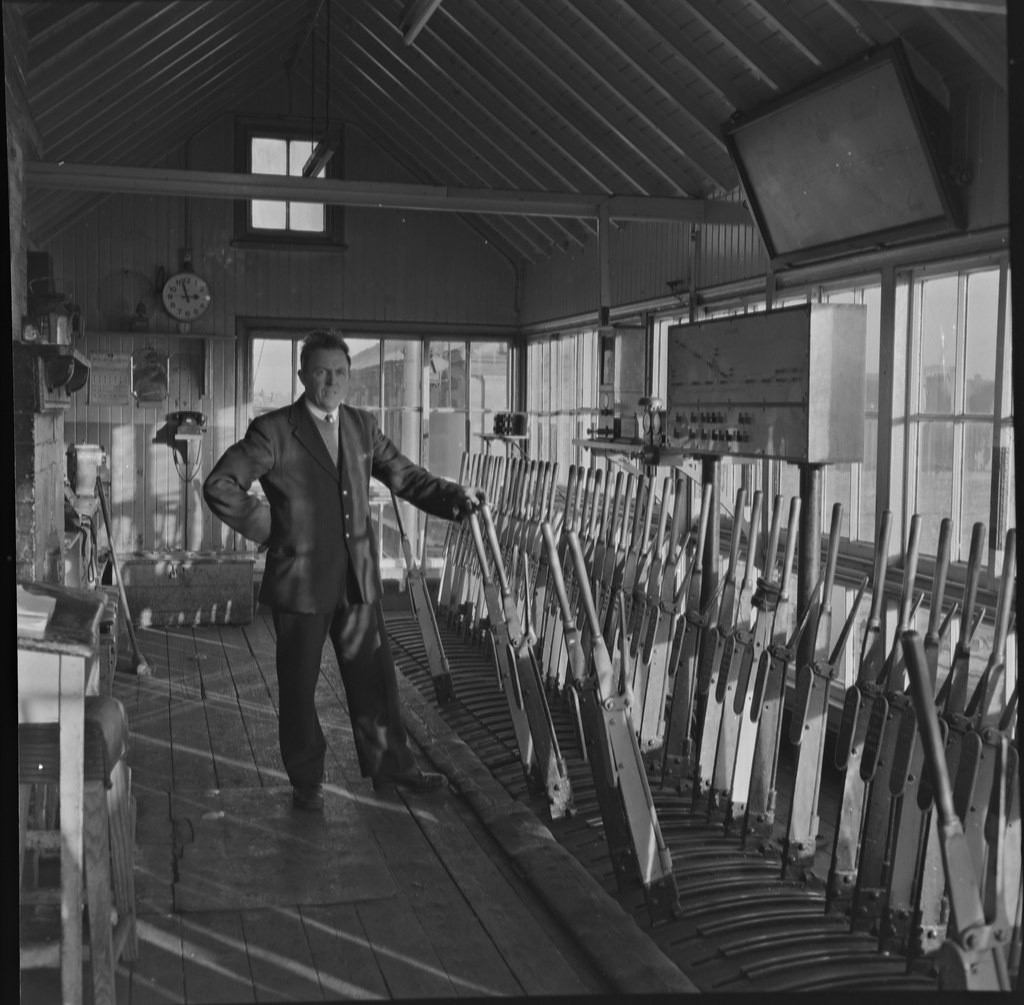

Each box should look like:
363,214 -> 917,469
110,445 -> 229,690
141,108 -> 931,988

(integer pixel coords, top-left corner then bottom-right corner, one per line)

122,550 -> 260,627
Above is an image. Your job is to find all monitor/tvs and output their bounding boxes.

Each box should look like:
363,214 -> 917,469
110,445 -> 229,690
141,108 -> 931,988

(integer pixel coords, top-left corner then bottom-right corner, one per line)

719,39 -> 962,273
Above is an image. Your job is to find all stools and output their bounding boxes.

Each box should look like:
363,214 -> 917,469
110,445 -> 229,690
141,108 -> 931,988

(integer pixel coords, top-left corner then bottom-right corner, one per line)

19,694 -> 141,1005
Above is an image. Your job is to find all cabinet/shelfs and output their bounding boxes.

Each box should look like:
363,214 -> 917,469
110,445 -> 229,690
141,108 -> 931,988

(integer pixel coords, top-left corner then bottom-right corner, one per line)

65,482 -> 124,690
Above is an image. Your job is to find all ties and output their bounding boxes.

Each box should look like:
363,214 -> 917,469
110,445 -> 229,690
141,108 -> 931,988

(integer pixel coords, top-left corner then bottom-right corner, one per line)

325,415 -> 334,423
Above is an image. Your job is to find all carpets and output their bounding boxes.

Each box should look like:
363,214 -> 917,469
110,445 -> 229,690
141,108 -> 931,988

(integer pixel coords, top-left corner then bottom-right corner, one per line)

169,785 -> 396,911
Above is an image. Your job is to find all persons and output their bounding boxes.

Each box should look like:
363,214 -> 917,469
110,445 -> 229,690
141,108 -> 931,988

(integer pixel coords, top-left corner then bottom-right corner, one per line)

204,333 -> 491,811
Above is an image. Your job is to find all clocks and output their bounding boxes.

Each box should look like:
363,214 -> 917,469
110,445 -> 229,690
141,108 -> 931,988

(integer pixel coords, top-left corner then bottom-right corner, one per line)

162,273 -> 212,323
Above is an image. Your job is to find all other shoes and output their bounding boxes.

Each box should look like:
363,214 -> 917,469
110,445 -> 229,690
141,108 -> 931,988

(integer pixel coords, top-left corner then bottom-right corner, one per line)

293,784 -> 325,810
372,772 -> 449,795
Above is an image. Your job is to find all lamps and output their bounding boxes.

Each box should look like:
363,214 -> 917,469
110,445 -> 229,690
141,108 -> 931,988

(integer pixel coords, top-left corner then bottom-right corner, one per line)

301,0 -> 339,179
396,0 -> 442,47
